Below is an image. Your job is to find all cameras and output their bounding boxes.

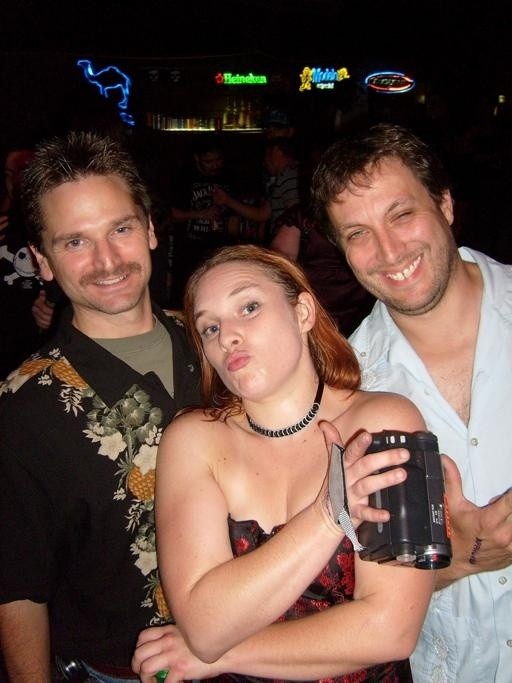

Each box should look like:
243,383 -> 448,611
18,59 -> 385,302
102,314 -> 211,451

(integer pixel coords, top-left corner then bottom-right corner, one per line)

358,429 -> 454,572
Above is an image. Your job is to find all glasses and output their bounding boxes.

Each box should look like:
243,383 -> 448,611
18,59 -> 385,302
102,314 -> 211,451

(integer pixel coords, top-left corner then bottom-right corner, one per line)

56,653 -> 99,683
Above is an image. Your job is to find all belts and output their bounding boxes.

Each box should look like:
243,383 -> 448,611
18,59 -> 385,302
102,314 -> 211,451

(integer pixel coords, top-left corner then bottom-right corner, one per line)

84,658 -> 140,679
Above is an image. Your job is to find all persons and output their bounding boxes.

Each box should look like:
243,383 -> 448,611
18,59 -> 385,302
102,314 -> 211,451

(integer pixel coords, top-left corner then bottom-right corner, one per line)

2,143 -> 509,370
130,243 -> 438,683
1,134 -> 234,683
308,123 -> 512,683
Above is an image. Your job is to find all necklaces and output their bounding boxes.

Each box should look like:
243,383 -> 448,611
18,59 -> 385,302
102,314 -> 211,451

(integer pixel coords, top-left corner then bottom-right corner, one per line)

242,373 -> 324,439
